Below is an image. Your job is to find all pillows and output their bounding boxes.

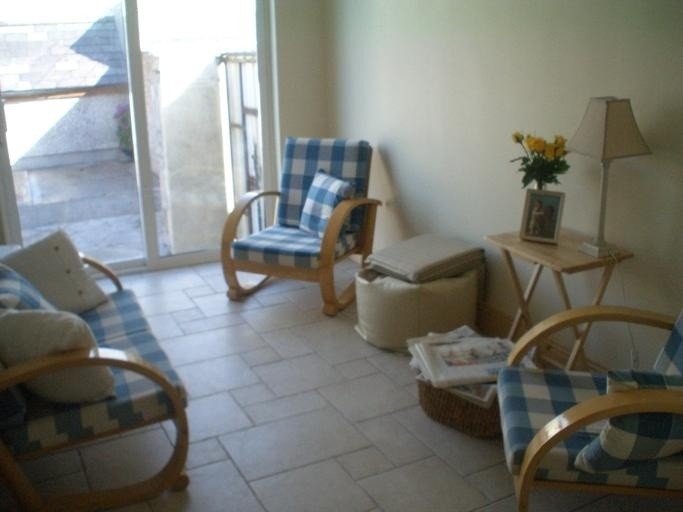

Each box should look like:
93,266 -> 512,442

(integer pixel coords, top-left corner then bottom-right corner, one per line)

0,308 -> 118,405
573,367 -> 683,474
0,262 -> 58,312
298,170 -> 357,244
0,226 -> 108,314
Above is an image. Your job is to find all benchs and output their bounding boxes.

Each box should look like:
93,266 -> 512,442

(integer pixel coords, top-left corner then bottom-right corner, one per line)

1,253 -> 190,512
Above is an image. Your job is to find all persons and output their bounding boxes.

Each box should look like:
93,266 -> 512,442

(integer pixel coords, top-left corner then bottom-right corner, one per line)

526,199 -> 545,235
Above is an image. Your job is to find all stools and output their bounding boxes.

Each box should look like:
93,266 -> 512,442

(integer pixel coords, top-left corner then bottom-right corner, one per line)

351,268 -> 481,353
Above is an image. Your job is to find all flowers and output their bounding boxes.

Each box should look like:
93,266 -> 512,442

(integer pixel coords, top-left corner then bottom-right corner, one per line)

508,130 -> 573,189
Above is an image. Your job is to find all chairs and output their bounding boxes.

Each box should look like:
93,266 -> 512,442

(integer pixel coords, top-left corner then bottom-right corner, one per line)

496,306 -> 683,511
219,137 -> 382,318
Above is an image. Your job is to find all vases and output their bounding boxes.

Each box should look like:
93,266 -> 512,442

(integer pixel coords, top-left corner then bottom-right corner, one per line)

534,182 -> 547,191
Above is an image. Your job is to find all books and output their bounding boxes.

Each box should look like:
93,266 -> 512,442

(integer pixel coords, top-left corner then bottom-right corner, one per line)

404,321 -> 544,411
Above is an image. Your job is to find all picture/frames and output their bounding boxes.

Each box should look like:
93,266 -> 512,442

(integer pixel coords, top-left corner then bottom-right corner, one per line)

520,189 -> 565,245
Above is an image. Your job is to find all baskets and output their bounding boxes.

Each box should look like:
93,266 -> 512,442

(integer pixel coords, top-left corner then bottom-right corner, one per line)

417,335 -> 543,442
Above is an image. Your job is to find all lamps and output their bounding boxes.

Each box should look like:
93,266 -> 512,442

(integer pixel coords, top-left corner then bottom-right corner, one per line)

566,95 -> 653,258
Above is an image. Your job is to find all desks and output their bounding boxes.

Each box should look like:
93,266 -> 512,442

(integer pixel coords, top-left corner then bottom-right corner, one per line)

484,225 -> 633,370
